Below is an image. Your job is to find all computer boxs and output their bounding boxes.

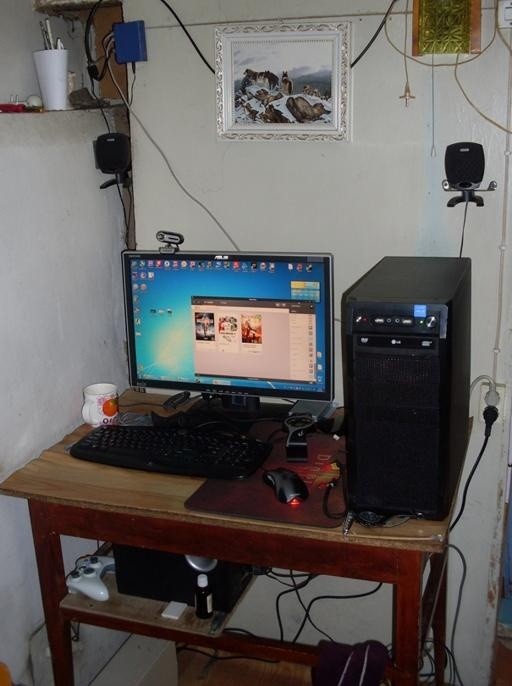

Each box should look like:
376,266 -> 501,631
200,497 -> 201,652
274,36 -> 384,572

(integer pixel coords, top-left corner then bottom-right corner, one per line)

340,256 -> 472,521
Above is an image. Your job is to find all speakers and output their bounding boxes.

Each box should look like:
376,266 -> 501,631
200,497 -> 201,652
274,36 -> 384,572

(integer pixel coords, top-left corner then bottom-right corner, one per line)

110,543 -> 252,613
92,132 -> 130,190
445,141 -> 484,207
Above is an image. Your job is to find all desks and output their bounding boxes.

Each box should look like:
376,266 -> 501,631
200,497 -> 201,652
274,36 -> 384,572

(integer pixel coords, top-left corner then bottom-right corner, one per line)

0,388 -> 474,686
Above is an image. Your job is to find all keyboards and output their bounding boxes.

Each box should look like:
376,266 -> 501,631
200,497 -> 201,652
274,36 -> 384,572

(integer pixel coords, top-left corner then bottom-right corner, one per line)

69,425 -> 272,481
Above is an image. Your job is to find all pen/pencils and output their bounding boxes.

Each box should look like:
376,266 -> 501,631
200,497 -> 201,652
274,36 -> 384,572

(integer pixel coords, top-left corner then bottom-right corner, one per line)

39,18 -> 64,50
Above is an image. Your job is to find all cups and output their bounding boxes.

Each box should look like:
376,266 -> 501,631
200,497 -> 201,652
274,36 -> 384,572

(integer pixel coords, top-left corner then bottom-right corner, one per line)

82,383 -> 119,428
31,49 -> 68,110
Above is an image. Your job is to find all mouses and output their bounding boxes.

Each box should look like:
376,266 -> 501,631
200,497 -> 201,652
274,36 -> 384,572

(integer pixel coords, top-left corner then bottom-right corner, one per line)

262,468 -> 309,504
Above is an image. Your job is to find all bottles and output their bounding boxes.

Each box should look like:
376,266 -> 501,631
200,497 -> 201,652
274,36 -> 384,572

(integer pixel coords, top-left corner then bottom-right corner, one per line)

195,574 -> 214,619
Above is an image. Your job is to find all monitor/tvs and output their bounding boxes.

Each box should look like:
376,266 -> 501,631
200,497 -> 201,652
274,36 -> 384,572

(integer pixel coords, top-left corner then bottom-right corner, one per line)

120,251 -> 335,435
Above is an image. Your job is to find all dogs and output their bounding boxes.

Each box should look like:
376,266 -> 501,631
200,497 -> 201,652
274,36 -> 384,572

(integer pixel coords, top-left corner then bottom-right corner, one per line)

242,68 -> 293,96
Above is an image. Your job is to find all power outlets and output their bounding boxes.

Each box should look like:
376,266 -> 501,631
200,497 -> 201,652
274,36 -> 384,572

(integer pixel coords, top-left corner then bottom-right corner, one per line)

479,383 -> 506,424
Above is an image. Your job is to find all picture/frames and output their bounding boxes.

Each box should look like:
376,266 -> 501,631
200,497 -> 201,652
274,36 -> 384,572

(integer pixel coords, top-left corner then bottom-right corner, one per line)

213,21 -> 352,143
